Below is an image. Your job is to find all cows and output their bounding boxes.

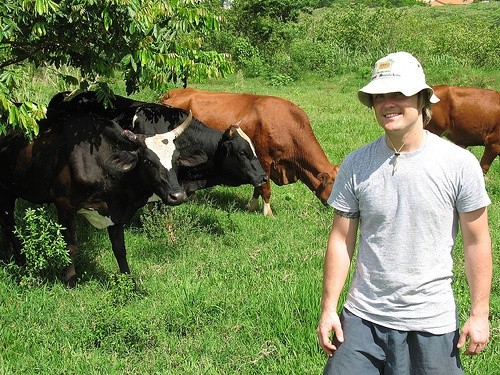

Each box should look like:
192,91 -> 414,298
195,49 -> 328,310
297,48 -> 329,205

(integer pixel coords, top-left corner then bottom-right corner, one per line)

0,110 -> 193,291
422,84 -> 500,176
158,87 -> 341,219
45,91 -> 270,240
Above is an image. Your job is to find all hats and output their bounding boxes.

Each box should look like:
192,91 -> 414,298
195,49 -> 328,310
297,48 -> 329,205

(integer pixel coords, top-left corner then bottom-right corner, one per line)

357,51 -> 440,109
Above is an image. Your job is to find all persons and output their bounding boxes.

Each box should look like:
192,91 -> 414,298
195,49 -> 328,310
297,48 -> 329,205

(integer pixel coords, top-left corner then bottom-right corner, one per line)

317,51 -> 492,375
